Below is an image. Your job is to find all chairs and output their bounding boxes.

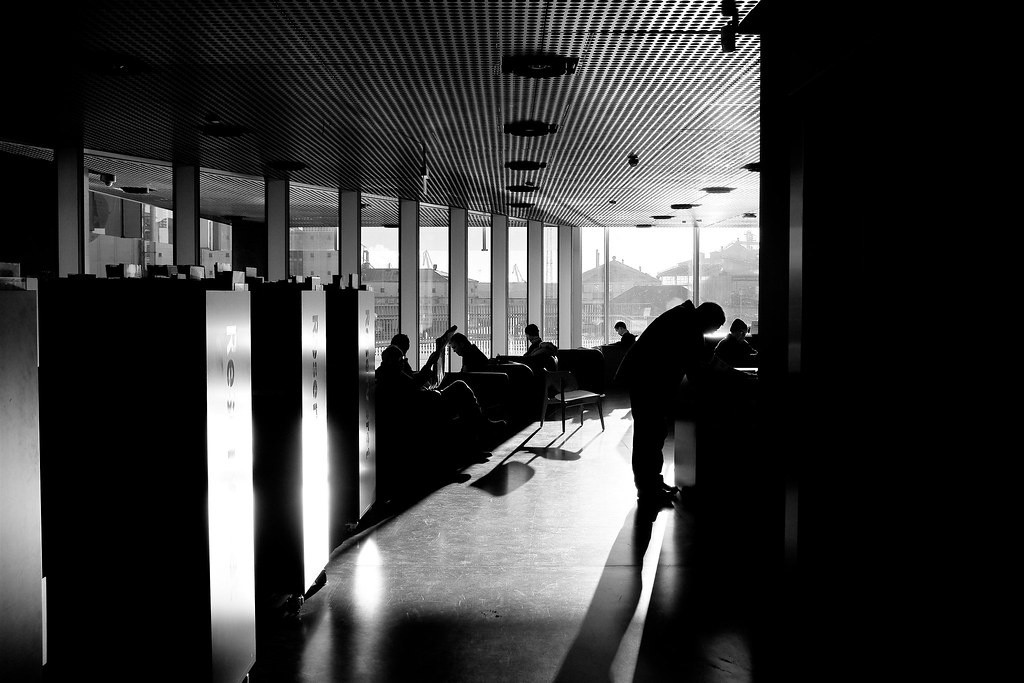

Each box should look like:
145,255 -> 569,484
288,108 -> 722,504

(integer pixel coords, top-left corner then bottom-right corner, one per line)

541,368 -> 606,434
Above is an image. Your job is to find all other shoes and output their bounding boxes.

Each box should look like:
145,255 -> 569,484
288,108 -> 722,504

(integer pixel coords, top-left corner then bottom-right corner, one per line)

638,479 -> 678,501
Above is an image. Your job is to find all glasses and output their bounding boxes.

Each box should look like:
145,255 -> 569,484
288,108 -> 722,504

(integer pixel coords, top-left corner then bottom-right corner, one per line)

733,331 -> 747,335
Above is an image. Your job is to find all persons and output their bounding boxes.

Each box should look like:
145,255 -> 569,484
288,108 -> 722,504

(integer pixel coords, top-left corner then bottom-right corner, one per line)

449,333 -> 493,372
522,324 -> 543,357
377,344 -> 507,441
616,300 -> 725,500
714,319 -> 753,368
529,341 -> 558,359
614,321 -> 637,346
390,334 -> 414,377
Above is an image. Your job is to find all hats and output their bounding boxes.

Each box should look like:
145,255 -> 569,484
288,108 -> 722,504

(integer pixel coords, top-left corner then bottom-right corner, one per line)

731,319 -> 746,331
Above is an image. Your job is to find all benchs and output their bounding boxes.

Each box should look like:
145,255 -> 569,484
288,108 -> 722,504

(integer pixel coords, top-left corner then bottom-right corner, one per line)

414,354 -> 559,422
557,340 -> 621,397
